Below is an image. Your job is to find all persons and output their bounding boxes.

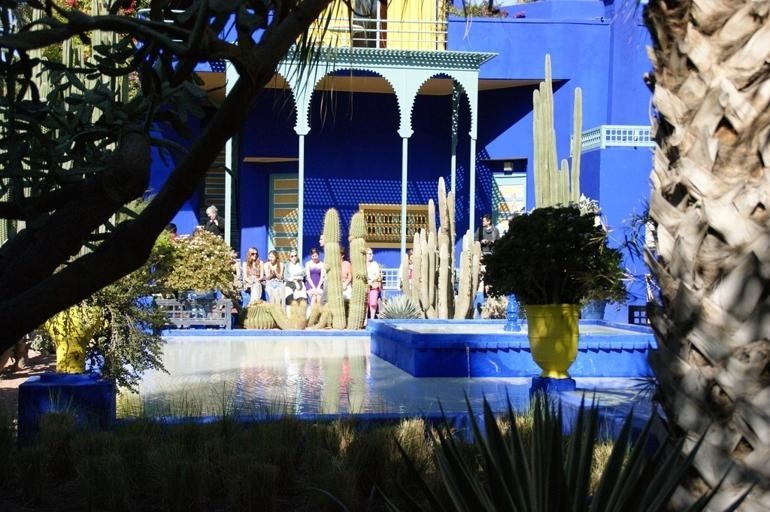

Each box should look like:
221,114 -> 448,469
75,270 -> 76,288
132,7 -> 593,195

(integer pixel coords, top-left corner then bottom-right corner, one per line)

203,204 -> 225,242
399,248 -> 413,290
476,214 -> 500,253
166,224 -> 182,242
241,246 -> 264,306
318,232 -> 327,305
339,244 -> 352,299
363,246 -> 384,327
284,249 -> 308,306
304,248 -> 327,305
263,250 -> 286,308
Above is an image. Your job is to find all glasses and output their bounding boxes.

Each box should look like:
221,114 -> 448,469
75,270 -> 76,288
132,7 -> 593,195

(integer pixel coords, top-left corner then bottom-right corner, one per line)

290,255 -> 297,258
250,253 -> 258,256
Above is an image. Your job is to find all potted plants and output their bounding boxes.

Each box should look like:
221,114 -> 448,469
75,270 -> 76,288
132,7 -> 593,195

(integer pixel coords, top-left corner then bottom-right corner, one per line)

484,199 -> 636,378
17,189 -> 238,450
